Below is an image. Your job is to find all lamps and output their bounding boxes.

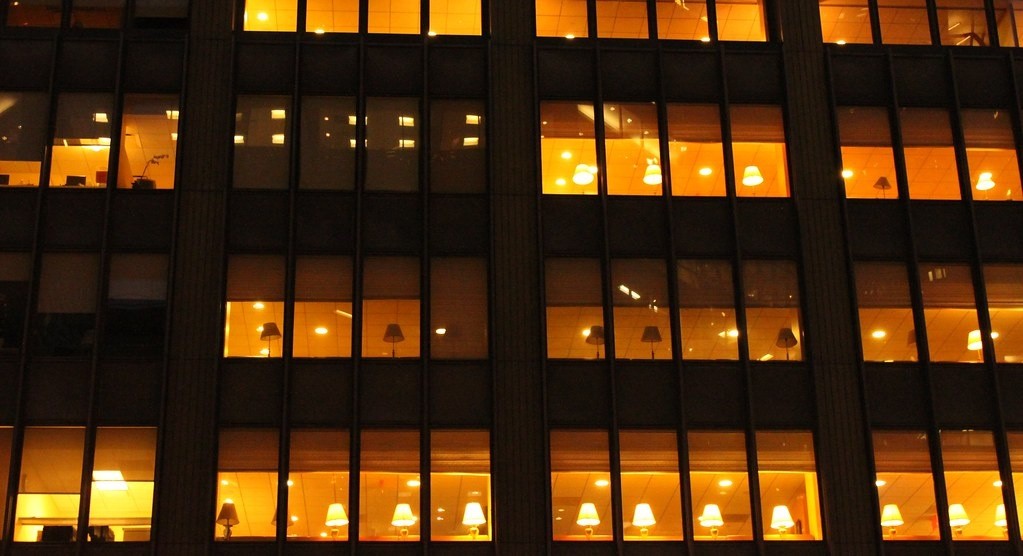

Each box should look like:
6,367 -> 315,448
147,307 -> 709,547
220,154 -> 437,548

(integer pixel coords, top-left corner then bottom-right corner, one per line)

212,159 -> 1009,539
